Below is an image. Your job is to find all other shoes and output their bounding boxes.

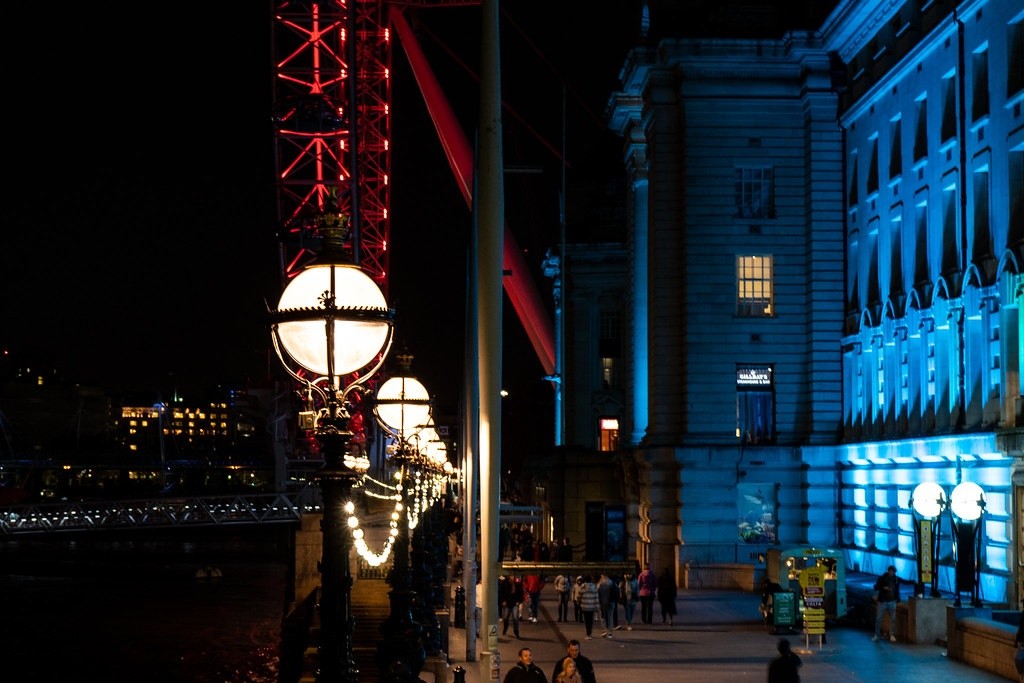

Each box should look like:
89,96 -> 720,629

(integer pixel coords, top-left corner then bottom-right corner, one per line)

890,637 -> 896,642
872,635 -> 878,642
615,626 -> 621,630
627,626 -> 632,631
601,632 -> 607,637
528,617 -> 538,623
609,634 -> 613,639
585,636 -> 590,640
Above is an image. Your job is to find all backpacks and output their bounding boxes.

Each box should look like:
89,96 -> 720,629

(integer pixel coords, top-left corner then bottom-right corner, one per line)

610,582 -> 620,602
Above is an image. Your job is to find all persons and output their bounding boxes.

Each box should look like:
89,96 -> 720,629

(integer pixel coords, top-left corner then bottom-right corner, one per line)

766,638 -> 803,683
441,520 -> 678,640
871,565 -> 916,642
804,590 -> 826,644
503,648 -> 549,683
760,578 -> 786,636
551,640 -> 597,683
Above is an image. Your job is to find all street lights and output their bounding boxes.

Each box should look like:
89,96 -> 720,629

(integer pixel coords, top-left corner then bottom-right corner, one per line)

405,413 -> 465,654
256,183 -> 401,683
151,402 -> 167,499
365,346 -> 434,680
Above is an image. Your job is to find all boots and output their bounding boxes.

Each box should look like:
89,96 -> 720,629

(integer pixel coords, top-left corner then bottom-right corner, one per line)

564,608 -> 568,622
557,609 -> 562,622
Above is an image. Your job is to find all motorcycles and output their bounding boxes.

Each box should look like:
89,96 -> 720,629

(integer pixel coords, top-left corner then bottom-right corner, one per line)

758,543 -> 849,635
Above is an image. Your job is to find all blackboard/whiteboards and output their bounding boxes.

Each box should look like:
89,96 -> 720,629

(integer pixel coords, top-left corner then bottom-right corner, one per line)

772,591 -> 796,625
